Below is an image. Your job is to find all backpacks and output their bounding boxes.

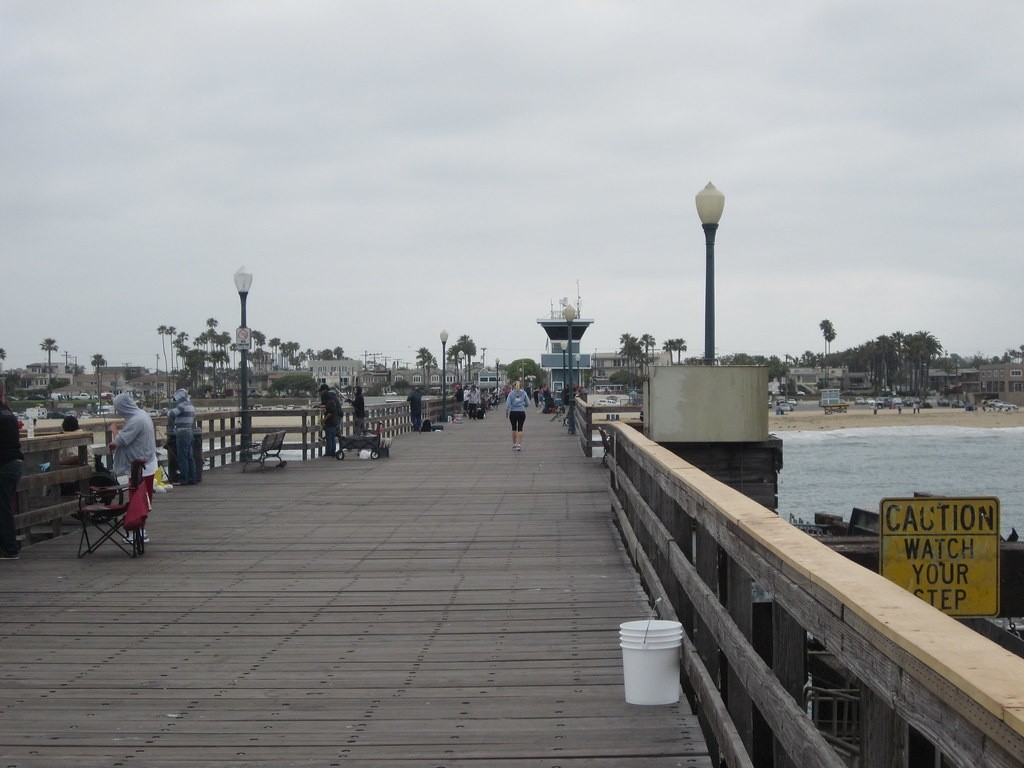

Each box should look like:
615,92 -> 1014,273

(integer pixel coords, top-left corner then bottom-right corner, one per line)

421,419 -> 432,433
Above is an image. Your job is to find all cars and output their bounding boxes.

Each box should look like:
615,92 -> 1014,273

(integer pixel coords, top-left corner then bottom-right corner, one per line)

276,404 -> 284,410
768,398 -> 797,411
286,404 -> 294,410
0,392 -> 161,419
986,398 -> 1018,410
210,392 -> 225,399
854,395 -> 932,410
937,399 -> 966,409
253,404 -> 262,411
598,399 -> 616,405
383,390 -> 397,396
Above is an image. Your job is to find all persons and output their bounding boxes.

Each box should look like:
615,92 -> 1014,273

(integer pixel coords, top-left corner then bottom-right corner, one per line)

454,385 -> 463,413
107,394 -> 156,543
163,389 -> 197,485
873,405 -> 878,414
897,404 -> 902,414
505,381 -> 530,451
463,386 -> 510,420
913,402 -> 920,413
314,383 -> 343,456
407,387 -> 422,430
57,392 -> 69,401
345,385 -> 364,436
40,416 -> 95,472
0,383 -> 24,559
526,383 -> 577,408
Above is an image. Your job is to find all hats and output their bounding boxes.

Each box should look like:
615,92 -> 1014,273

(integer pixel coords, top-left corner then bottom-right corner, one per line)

318,384 -> 330,392
63,416 -> 79,432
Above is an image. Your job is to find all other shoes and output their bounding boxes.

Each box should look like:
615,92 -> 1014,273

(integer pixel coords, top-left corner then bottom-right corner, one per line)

172,479 -> 196,486
323,453 -> 337,457
0,550 -> 20,560
517,445 -> 520,451
512,445 -> 516,451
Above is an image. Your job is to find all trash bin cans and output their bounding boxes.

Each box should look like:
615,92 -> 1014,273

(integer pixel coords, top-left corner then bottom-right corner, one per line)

545,397 -> 554,406
165,429 -> 203,482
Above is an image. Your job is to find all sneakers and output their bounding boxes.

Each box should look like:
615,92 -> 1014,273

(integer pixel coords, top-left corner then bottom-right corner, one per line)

122,531 -> 150,544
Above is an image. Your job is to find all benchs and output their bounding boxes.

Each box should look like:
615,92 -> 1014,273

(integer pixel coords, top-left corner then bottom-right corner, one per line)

241,430 -> 286,472
597,425 -> 611,464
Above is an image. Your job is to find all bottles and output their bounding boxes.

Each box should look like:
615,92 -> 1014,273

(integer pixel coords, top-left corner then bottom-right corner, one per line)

27,420 -> 35,439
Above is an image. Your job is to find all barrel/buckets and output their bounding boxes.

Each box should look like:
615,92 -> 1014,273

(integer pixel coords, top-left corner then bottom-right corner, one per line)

619,598 -> 684,706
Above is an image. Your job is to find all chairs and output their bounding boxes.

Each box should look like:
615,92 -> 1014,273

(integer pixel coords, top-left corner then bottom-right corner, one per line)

70,456 -> 147,558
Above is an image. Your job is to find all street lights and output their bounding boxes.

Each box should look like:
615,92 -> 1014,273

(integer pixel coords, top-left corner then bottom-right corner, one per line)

494,357 -> 500,405
439,328 -> 448,423
232,265 -> 253,461
561,304 -> 577,434
694,180 -> 726,366
